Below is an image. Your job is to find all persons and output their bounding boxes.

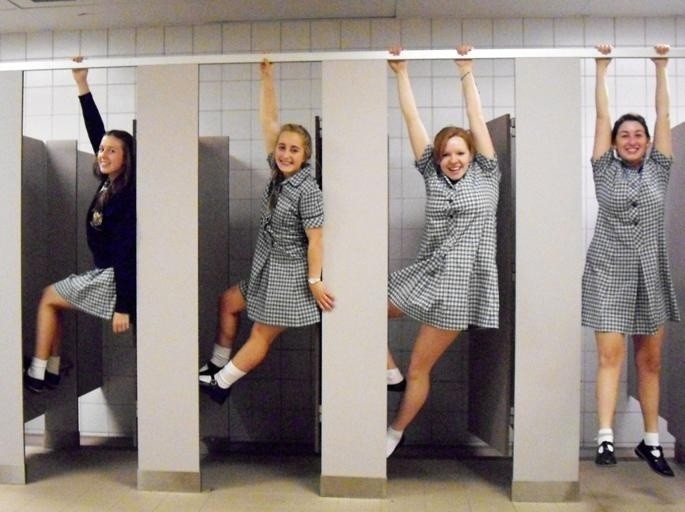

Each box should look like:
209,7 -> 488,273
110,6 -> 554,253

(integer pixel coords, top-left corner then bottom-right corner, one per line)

386,45 -> 503,460
23,55 -> 135,396
581,43 -> 683,478
200,58 -> 336,406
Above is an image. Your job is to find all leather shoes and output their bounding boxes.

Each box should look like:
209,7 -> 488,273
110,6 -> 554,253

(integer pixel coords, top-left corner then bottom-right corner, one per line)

199,359 -> 228,375
199,373 -> 231,406
387,377 -> 407,394
23,370 -> 46,395
634,438 -> 675,479
23,361 -> 62,391
386,427 -> 404,461
592,438 -> 618,466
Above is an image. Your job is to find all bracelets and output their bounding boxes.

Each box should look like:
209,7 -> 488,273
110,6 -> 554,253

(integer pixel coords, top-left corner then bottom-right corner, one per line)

308,278 -> 322,283
460,70 -> 472,82
